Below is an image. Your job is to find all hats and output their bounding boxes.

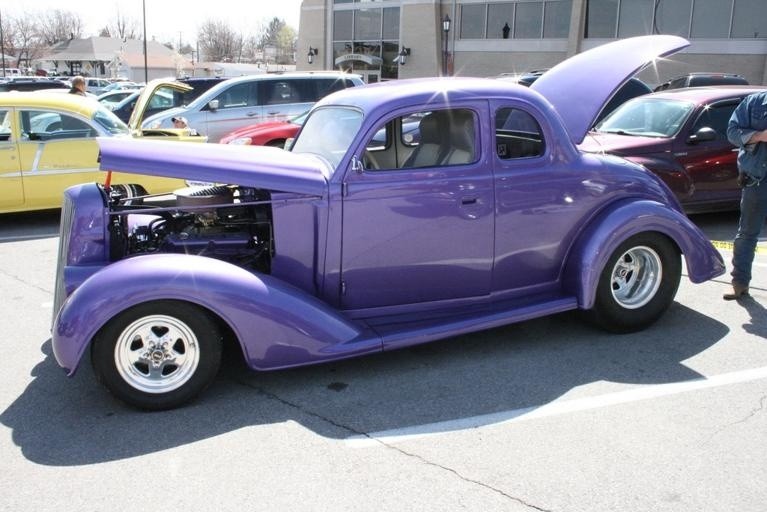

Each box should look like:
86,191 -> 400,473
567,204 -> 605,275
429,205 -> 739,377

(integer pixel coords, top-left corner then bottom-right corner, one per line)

172,117 -> 187,126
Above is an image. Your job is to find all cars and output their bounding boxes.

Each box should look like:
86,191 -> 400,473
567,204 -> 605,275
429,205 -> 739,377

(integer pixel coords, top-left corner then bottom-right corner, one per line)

45,32 -> 727,412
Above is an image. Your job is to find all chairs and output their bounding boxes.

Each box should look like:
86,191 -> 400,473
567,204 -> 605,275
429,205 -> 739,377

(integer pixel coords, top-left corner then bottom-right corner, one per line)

399,113 -> 473,170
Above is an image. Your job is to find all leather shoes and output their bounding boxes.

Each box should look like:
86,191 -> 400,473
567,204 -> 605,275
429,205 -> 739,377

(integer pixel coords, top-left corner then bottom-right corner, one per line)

723,282 -> 749,299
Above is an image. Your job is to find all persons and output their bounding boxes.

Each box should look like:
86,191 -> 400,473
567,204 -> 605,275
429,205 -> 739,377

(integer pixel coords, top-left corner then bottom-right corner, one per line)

724,91 -> 767,300
502,22 -> 510,39
171,117 -> 200,137
68,76 -> 87,96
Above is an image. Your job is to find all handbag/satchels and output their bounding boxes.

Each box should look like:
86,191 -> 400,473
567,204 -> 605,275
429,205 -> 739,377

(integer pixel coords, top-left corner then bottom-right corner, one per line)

737,172 -> 751,189
